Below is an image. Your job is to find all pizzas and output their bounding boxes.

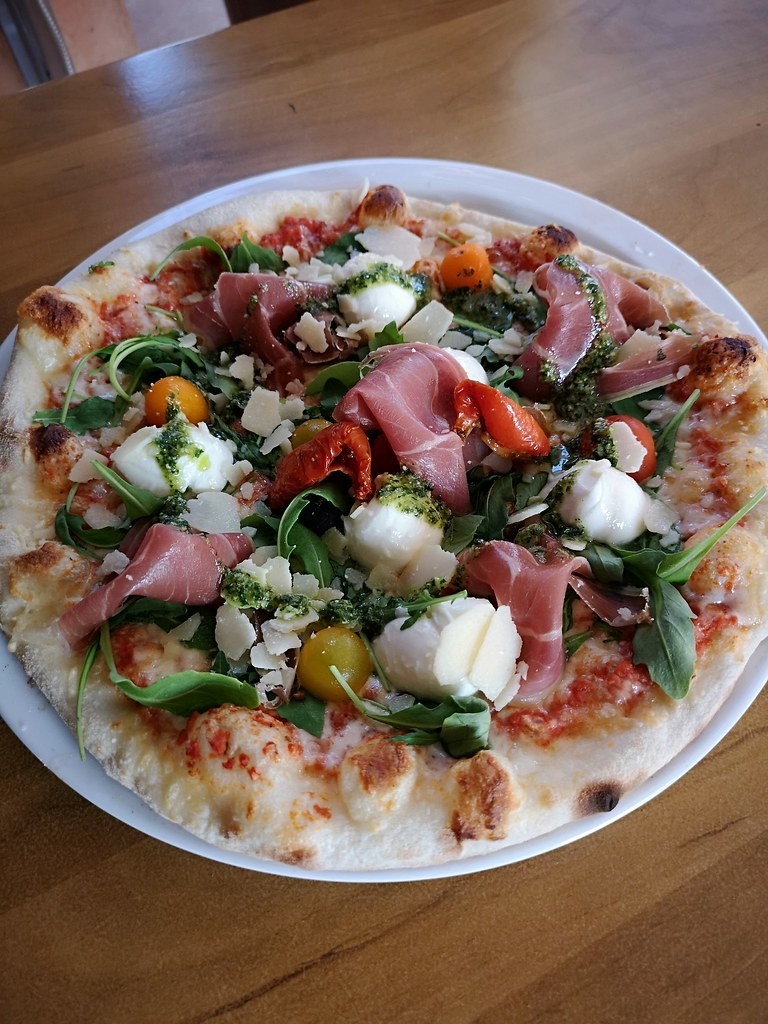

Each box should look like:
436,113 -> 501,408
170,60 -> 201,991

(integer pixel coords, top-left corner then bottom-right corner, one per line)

0,182 -> 768,873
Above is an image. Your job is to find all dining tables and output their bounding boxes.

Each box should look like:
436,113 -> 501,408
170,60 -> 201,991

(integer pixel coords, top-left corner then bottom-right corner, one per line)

0,0 -> 768,1024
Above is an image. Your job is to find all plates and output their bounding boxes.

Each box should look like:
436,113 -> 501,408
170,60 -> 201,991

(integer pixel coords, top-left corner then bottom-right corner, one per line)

1,158 -> 767,885
